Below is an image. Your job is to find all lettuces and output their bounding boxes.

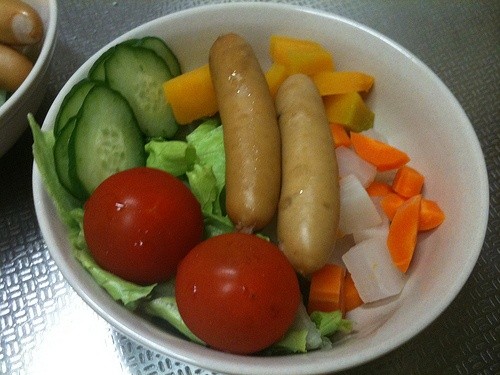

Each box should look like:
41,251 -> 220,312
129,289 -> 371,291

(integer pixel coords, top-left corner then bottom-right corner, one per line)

27,111 -> 354,353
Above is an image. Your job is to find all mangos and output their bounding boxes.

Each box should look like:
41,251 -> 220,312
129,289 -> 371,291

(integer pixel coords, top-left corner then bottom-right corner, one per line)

161,35 -> 379,133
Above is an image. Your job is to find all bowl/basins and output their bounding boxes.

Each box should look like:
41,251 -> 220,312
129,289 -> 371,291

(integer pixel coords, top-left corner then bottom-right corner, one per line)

0,0 -> 58,155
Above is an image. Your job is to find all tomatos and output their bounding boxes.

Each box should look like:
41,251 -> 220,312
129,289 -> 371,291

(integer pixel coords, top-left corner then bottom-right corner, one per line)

84,167 -> 299,355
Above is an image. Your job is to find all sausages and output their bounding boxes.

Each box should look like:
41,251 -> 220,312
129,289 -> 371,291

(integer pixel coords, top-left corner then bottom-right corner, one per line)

211,32 -> 341,274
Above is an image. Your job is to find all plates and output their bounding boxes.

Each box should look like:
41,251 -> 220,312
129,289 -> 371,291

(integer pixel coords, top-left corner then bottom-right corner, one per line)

33,1 -> 490,375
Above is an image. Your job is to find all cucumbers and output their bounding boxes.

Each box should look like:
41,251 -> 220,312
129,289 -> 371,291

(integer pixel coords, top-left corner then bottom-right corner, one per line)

52,37 -> 185,200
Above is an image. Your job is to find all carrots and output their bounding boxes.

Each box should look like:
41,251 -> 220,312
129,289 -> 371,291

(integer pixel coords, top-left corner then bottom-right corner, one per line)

308,123 -> 446,316
